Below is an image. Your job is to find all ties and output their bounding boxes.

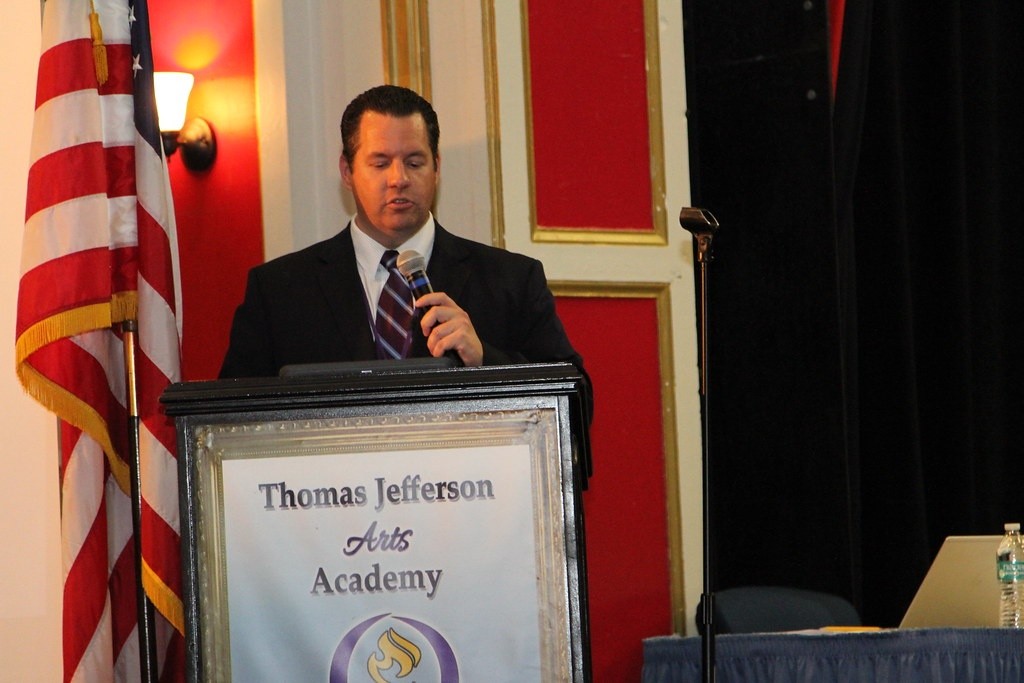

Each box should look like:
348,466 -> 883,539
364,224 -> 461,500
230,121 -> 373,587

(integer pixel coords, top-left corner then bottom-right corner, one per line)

373,249 -> 415,360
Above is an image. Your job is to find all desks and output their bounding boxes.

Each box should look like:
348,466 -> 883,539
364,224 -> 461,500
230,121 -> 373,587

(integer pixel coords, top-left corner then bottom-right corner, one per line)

641,627 -> 1024,683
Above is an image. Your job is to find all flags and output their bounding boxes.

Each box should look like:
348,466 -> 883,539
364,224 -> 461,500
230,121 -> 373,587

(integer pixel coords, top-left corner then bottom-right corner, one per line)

14,0 -> 186,683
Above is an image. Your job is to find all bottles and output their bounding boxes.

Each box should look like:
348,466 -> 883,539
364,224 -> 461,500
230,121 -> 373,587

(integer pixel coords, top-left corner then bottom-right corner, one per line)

995,524 -> 1024,628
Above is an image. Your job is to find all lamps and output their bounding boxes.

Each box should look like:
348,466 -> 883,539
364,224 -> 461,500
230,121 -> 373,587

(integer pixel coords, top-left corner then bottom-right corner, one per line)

152,71 -> 216,172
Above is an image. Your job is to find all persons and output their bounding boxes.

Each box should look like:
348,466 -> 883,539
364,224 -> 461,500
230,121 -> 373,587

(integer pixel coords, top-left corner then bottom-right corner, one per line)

217,84 -> 595,424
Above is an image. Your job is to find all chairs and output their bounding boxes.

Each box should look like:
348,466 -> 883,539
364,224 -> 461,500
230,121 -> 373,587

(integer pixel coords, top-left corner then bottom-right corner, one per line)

692,587 -> 860,635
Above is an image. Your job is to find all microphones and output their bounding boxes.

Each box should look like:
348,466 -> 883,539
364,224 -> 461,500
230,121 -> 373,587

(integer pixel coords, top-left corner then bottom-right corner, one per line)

397,250 -> 465,368
